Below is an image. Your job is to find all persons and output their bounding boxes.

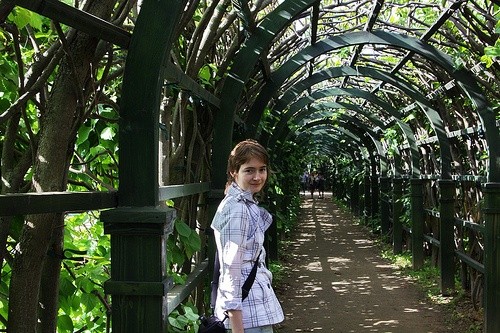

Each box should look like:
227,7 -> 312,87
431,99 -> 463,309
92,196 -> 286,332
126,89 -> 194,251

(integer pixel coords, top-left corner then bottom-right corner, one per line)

299,169 -> 327,199
209,138 -> 285,333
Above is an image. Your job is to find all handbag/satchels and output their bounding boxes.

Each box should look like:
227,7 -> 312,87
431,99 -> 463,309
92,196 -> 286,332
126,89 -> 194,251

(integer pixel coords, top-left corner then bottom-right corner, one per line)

196,314 -> 227,333
